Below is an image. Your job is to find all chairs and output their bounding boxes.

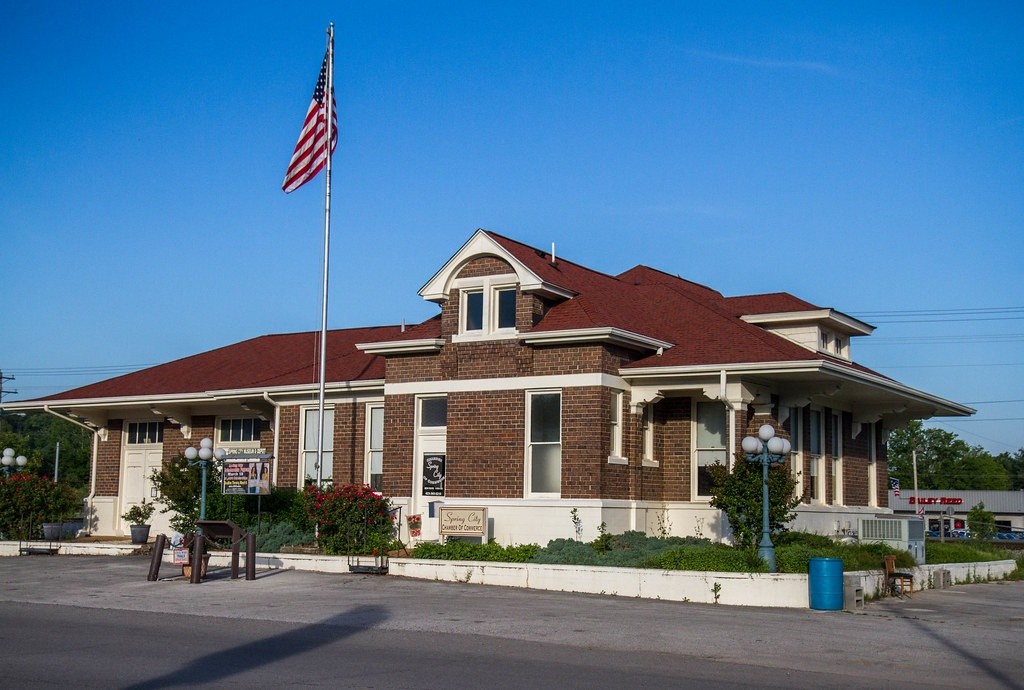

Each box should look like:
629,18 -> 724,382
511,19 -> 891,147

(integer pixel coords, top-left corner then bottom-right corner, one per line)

883,555 -> 914,601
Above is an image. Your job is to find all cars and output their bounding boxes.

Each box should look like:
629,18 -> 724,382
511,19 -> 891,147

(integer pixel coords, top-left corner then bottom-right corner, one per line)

925,530 -> 1024,540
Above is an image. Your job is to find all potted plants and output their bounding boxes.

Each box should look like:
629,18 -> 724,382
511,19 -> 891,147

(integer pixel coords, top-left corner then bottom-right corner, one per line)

146,441 -> 255,580
122,498 -> 155,543
42,482 -> 78,540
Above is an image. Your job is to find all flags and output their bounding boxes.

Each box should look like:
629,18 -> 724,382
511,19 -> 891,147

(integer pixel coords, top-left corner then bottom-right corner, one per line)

280,33 -> 339,195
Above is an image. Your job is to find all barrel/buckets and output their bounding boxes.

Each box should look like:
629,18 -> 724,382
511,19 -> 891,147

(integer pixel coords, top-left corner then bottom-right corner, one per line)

808,557 -> 844,611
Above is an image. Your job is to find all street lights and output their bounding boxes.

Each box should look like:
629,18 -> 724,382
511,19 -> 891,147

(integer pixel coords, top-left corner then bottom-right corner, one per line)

184,436 -> 226,584
912,446 -> 925,515
741,424 -> 792,573
